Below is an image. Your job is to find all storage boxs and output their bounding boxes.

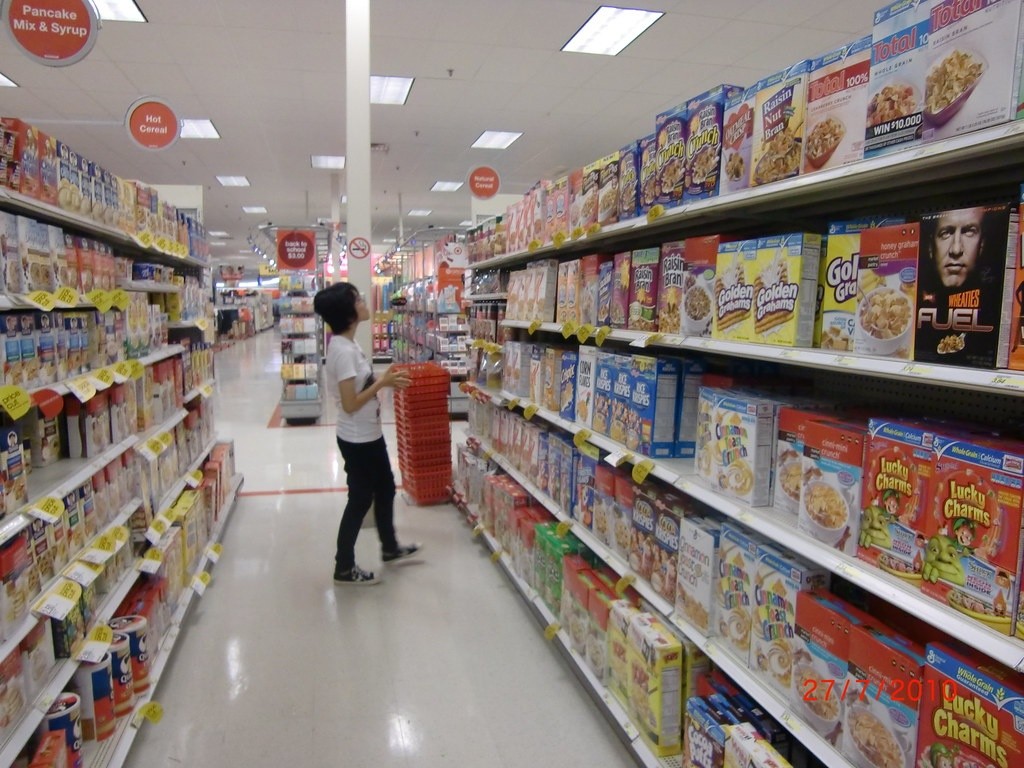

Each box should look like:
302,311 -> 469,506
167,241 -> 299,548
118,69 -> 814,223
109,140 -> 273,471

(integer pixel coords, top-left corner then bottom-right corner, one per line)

0,113 -> 235,768
451,0 -> 1024,768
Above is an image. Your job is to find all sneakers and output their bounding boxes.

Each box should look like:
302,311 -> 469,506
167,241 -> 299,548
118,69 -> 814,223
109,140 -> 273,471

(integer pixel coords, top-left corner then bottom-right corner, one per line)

334,564 -> 380,585
383,542 -> 423,565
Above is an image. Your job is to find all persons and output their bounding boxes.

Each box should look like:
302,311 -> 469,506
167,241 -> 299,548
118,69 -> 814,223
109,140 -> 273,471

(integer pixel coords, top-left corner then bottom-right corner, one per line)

313,281 -> 424,587
917,206 -> 997,364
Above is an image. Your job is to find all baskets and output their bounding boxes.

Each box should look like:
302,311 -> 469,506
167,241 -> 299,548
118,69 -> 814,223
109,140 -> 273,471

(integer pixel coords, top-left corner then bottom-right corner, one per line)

393,363 -> 453,500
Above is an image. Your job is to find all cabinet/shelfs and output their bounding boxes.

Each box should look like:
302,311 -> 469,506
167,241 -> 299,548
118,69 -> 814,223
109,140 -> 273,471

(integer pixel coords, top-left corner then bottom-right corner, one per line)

320,276 -> 392,364
276,273 -> 322,425
441,114 -> 1024,768
213,287 -> 273,350
0,184 -> 245,768
384,224 -> 469,420
